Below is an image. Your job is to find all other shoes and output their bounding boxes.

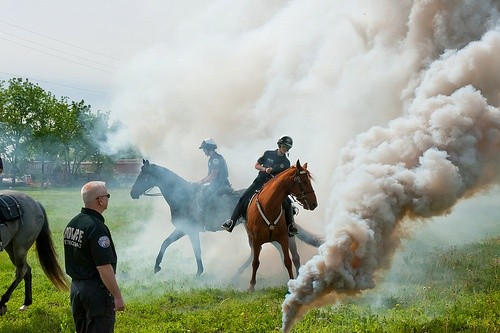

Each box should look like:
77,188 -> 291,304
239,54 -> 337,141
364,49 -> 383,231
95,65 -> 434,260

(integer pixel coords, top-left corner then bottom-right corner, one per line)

222,215 -> 236,233
287,222 -> 299,238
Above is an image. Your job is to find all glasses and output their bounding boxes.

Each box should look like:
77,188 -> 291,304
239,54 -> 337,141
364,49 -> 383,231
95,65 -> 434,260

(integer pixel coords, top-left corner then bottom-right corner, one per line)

96,194 -> 110,200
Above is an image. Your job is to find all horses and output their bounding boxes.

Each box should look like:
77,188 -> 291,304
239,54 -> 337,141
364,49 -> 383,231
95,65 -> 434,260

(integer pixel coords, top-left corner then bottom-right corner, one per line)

131,159 -> 285,288
0,190 -> 72,315
246,160 -> 325,293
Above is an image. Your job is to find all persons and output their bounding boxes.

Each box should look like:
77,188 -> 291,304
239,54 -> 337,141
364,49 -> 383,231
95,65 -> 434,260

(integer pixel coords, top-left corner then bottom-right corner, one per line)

192,138 -> 231,232
62,180 -> 126,333
223,135 -> 298,238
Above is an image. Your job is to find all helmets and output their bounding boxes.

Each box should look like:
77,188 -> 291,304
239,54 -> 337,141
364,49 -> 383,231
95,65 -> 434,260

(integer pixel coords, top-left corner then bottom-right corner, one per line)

277,136 -> 293,147
199,137 -> 217,150
